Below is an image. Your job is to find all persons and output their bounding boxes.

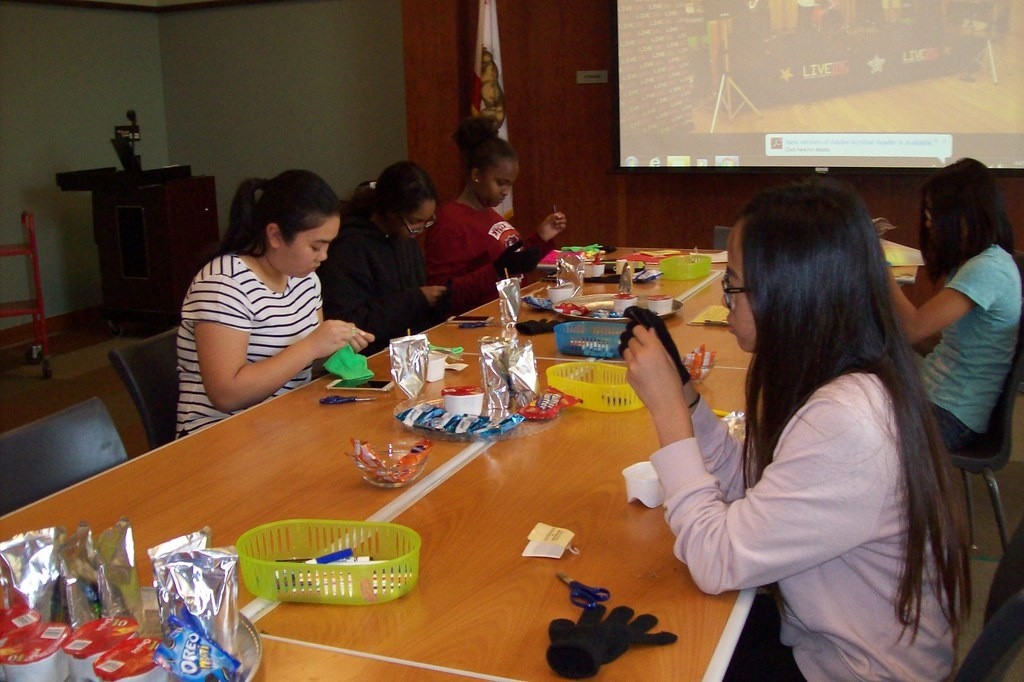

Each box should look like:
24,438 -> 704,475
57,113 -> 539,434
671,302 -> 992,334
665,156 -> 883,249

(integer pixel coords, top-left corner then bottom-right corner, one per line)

878,157 -> 1023,452
422,117 -> 568,315
315,160 -> 448,358
618,175 -> 970,682
175,168 -> 375,439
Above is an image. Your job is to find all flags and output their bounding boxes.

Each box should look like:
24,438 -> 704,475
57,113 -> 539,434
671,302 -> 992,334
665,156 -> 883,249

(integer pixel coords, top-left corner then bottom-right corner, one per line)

470,0 -> 513,223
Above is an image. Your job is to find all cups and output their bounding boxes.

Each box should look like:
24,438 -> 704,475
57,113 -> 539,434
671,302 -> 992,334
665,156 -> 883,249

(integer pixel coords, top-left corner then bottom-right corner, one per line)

646,295 -> 674,315
621,461 -> 665,508
613,259 -> 629,274
426,354 -> 447,382
546,284 -> 575,304
0,606 -> 169,682
441,386 -> 486,416
610,293 -> 639,312
585,264 -> 605,277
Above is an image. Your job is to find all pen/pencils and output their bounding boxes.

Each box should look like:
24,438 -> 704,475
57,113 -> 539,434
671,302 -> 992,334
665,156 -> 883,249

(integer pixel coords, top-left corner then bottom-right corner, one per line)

271,554 -> 414,596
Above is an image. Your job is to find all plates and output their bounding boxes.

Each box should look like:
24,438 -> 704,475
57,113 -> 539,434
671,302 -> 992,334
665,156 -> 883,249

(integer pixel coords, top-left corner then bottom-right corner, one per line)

392,392 -> 561,441
0,587 -> 262,682
551,293 -> 684,323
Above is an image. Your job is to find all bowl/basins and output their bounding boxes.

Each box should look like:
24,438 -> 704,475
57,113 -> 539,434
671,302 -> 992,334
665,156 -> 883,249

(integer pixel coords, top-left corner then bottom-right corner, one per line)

356,441 -> 429,487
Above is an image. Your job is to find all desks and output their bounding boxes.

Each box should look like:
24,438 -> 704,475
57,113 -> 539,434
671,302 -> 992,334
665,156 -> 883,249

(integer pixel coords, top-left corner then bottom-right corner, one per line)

0,246 -> 757,682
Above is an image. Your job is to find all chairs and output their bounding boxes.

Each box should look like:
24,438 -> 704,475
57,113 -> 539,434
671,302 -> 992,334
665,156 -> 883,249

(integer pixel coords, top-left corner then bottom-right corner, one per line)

107,326 -> 179,452
0,397 -> 128,521
947,247 -> 1024,553
952,519 -> 1024,682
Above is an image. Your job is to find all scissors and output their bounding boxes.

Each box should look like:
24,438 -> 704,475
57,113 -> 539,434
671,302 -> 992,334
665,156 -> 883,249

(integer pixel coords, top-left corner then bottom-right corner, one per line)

557,571 -> 611,609
459,322 -> 502,328
319,394 -> 378,404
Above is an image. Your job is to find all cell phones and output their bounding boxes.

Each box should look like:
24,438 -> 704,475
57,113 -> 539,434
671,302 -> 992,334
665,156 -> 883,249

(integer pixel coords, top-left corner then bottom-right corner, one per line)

447,316 -> 494,323
326,379 -> 394,391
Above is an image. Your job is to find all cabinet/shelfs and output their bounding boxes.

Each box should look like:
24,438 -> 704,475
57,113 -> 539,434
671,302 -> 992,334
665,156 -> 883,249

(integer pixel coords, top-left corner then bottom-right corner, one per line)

0,211 -> 52,379
92,175 -> 220,339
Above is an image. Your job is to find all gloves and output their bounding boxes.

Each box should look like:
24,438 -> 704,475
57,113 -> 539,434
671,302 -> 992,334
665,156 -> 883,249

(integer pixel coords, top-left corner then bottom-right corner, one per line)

618,306 -> 692,387
516,319 -> 559,335
494,242 -> 539,278
547,604 -> 677,680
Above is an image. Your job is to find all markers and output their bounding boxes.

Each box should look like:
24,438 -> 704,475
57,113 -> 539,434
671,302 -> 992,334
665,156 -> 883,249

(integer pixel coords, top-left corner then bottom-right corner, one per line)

302,548 -> 355,568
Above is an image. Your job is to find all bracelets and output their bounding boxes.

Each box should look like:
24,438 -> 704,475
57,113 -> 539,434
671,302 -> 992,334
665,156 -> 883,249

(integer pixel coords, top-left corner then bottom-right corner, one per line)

688,393 -> 700,408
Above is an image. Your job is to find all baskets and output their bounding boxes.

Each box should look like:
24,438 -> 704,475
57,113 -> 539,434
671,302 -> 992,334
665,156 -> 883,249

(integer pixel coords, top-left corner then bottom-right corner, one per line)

553,320 -> 626,357
236,519 -> 421,605
660,255 -> 711,280
545,360 -> 645,412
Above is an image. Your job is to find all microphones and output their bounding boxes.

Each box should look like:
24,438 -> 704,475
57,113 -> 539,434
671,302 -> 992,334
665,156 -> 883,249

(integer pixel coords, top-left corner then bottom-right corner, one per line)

126,109 -> 137,126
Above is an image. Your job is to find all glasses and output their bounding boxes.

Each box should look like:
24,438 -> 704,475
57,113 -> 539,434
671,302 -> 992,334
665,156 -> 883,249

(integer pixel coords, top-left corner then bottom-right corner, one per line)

721,276 -> 751,307
397,213 -> 437,234
